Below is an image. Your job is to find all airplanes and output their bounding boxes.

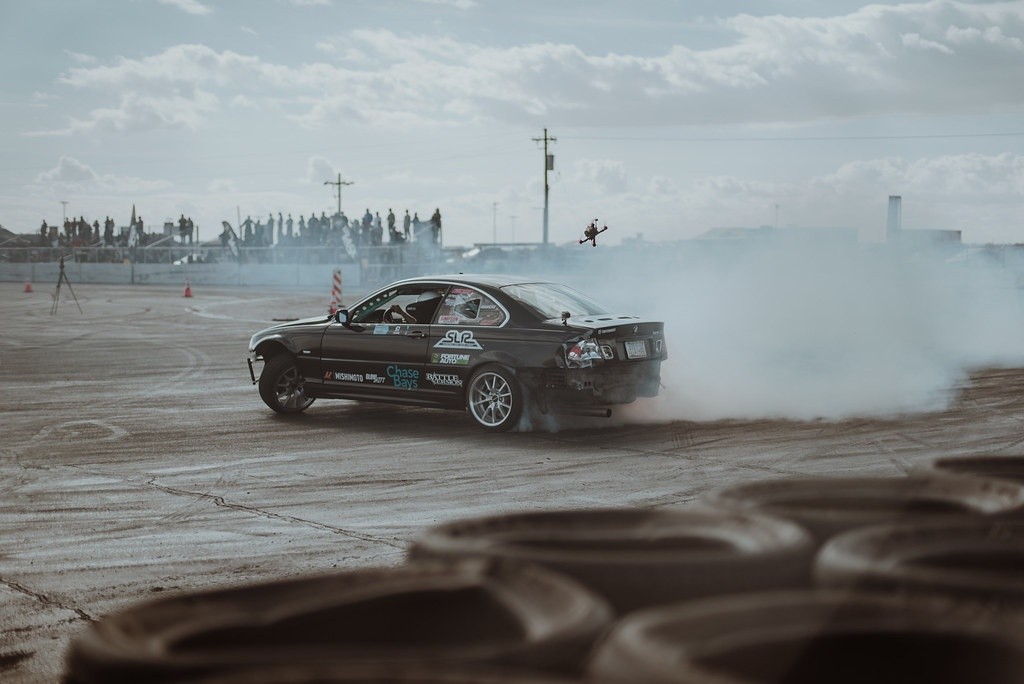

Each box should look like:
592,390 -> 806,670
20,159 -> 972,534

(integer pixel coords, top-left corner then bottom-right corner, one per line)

579,218 -> 608,247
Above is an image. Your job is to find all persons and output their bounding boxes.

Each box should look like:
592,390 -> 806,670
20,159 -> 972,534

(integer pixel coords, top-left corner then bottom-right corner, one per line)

40,209 -> 443,246
380,291 -> 443,325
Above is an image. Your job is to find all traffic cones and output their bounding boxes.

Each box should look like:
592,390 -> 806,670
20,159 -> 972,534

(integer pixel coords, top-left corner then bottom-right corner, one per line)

21,277 -> 33,293
181,274 -> 193,298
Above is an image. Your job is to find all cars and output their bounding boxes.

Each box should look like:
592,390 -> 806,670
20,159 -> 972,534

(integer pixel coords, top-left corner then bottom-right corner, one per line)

247,271 -> 669,432
